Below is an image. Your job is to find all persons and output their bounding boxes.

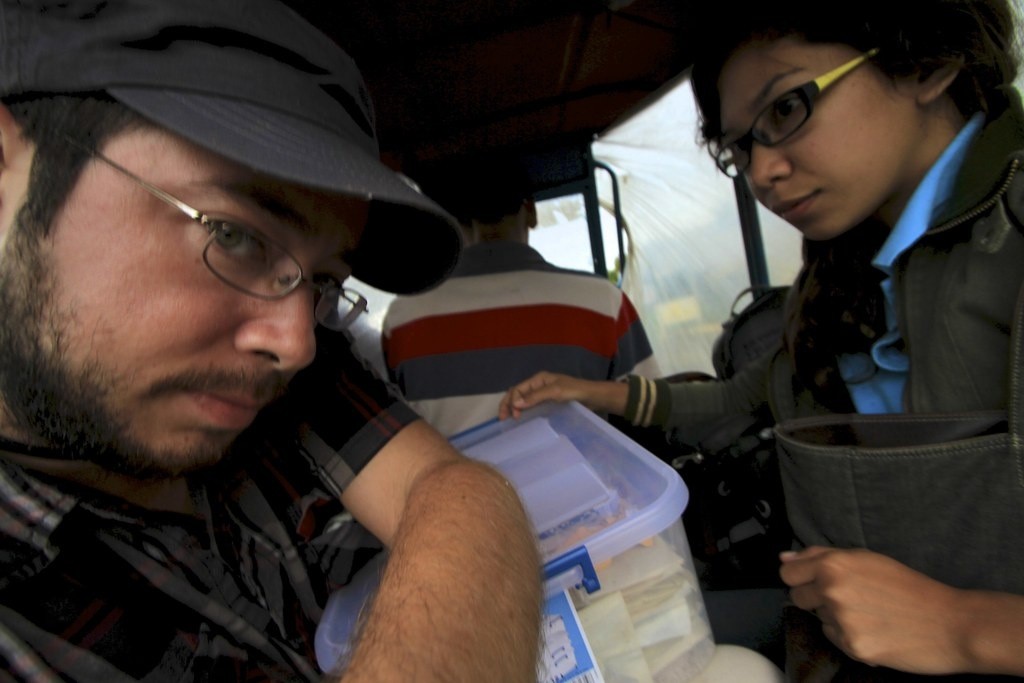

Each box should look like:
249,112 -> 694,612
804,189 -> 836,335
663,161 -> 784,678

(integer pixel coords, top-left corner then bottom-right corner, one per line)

381,142 -> 652,399
0,0 -> 541,683
495,0 -> 1024,683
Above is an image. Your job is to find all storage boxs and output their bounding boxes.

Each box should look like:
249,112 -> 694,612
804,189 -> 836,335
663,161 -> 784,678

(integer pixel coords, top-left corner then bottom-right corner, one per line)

314,397 -> 715,683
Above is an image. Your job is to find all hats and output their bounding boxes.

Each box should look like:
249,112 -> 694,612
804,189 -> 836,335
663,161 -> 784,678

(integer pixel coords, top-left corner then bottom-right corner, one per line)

0,0 -> 463,297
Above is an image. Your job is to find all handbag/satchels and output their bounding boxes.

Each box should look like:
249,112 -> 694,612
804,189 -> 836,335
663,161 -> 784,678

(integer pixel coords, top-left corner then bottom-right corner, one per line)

711,282 -> 795,379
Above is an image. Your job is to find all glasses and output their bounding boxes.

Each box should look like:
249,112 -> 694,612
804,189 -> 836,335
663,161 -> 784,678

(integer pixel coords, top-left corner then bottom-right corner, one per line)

715,42 -> 898,178
31,119 -> 368,330
